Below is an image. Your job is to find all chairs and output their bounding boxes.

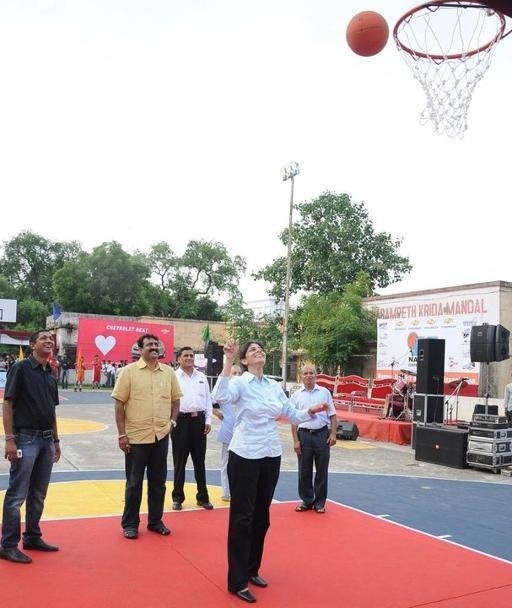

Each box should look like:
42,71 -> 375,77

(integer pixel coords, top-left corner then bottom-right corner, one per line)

316,374 -> 414,422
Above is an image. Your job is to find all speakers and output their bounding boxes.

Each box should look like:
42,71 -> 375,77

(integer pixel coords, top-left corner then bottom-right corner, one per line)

414,426 -> 468,469
416,338 -> 445,395
470,324 -> 510,363
207,345 -> 223,376
334,422 -> 359,441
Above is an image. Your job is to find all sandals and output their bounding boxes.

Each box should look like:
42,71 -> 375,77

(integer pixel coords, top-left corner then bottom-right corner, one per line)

296,501 -> 313,511
314,501 -> 326,513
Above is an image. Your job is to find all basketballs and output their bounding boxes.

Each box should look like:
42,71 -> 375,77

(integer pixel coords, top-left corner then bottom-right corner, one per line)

347,11 -> 390,55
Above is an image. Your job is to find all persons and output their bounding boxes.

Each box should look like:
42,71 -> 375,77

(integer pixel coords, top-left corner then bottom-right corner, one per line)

0,353 -> 137,392
0,328 -> 61,563
110,333 -> 184,539
211,364 -> 242,502
215,338 -> 331,602
170,346 -> 213,509
289,363 -> 338,513
503,372 -> 512,428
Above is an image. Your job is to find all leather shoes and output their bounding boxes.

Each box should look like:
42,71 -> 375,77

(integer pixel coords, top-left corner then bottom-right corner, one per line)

249,574 -> 268,587
23,536 -> 59,551
0,543 -> 32,563
197,500 -> 214,510
237,587 -> 256,603
123,522 -> 139,539
147,522 -> 171,536
171,500 -> 183,510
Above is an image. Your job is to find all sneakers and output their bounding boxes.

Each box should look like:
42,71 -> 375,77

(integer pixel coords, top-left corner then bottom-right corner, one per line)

221,495 -> 230,502
61,384 -> 101,393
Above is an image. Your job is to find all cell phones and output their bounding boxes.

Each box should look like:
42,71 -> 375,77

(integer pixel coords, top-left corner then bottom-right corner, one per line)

17,449 -> 23,459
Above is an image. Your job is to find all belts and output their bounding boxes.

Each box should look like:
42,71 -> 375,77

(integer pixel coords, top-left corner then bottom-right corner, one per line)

176,410 -> 208,418
16,428 -> 54,439
298,426 -> 328,434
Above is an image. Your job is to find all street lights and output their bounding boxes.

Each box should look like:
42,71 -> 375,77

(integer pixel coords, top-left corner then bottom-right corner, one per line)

281,159 -> 299,395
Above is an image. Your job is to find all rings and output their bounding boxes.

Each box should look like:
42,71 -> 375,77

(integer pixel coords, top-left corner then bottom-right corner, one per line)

125,444 -> 129,447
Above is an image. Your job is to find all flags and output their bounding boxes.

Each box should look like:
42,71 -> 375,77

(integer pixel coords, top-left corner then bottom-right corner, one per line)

53,301 -> 62,321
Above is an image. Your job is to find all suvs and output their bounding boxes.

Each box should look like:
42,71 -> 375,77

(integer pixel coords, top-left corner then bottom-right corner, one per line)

132,339 -> 166,360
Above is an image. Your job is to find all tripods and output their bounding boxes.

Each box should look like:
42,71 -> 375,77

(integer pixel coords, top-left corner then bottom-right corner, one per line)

396,396 -> 412,421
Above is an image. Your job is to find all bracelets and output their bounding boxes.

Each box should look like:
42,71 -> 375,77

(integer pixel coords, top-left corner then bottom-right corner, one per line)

54,438 -> 59,443
309,408 -> 316,415
171,418 -> 178,428
4,434 -> 14,441
117,433 -> 126,437
